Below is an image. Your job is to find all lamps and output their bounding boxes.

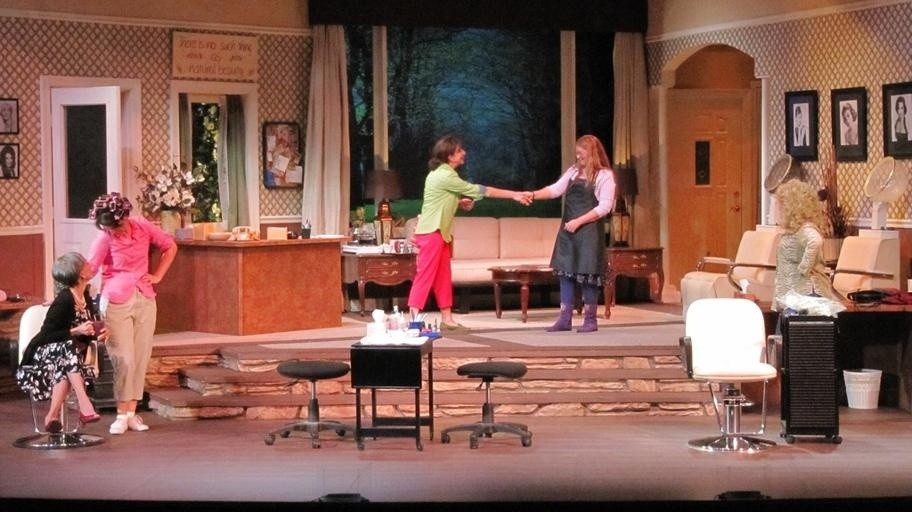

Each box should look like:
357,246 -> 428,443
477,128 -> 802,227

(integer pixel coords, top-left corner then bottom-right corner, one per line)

363,169 -> 407,244
614,161 -> 640,247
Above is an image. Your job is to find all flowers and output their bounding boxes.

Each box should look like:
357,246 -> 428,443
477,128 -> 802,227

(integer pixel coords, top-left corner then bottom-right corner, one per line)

130,163 -> 213,215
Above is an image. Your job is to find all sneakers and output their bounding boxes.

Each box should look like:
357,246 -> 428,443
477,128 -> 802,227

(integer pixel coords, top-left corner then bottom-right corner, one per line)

439,322 -> 469,332
110,412 -> 149,434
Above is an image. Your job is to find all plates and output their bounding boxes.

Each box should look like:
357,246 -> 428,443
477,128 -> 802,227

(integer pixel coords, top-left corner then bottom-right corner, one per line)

419,332 -> 440,338
207,233 -> 232,241
846,289 -> 888,301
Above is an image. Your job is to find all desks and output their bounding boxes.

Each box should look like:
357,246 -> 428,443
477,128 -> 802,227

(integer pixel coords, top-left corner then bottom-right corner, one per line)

0,294 -> 47,369
776,308 -> 912,421
606,247 -> 664,306
350,337 -> 434,455
342,253 -> 418,316
150,237 -> 344,336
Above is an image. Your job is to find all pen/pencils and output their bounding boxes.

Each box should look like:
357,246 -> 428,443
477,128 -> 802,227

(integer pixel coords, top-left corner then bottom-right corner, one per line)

411,309 -> 428,322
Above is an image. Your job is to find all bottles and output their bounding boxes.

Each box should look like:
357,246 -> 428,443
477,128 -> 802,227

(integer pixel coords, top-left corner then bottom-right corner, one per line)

239,228 -> 250,241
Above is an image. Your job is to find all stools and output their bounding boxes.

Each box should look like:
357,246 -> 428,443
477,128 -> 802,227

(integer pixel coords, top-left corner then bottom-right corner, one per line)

440,360 -> 536,451
264,360 -> 366,453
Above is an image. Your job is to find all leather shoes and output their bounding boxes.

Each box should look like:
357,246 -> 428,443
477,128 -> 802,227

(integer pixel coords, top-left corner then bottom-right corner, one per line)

46,419 -> 62,434
80,411 -> 99,423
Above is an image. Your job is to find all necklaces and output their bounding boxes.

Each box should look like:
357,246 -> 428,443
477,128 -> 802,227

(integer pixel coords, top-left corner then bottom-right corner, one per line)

69,287 -> 86,304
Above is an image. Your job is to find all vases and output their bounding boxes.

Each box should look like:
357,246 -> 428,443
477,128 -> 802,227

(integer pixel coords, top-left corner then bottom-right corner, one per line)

159,209 -> 181,234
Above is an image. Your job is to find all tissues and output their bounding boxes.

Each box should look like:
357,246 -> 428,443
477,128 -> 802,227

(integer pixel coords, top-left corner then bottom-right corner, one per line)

365,308 -> 388,343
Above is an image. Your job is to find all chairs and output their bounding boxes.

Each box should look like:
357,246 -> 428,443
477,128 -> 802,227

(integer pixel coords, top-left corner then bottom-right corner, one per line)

678,222 -> 786,326
826,235 -> 901,297
11,305 -> 104,450
678,296 -> 781,454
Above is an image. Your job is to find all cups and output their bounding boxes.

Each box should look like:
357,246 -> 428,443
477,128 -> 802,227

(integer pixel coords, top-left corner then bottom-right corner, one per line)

93,321 -> 105,337
301,228 -> 311,240
409,321 -> 426,331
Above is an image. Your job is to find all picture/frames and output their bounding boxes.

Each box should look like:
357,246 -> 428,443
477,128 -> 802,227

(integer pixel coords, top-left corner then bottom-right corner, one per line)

829,86 -> 869,164
882,80 -> 912,161
0,98 -> 20,136
262,119 -> 305,190
0,142 -> 21,181
784,89 -> 820,163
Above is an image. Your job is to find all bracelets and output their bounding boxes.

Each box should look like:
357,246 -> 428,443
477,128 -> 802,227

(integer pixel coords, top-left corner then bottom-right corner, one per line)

533,191 -> 534,200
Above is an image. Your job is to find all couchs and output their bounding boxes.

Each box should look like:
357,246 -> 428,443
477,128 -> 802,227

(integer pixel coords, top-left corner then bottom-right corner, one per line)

405,215 -> 561,306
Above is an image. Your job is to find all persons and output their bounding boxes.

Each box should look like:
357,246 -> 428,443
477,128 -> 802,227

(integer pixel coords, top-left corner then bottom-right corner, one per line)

406,135 -> 534,332
842,103 -> 858,145
892,96 -> 912,142
793,106 -> 809,147
522,135 -> 617,333
0,146 -> 15,177
0,103 -> 17,132
16,252 -> 111,434
87,192 -> 179,435
769,178 -> 832,361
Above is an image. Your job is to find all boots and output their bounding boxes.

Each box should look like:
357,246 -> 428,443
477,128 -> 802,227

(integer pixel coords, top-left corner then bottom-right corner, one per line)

545,303 -> 573,332
576,303 -> 598,332
